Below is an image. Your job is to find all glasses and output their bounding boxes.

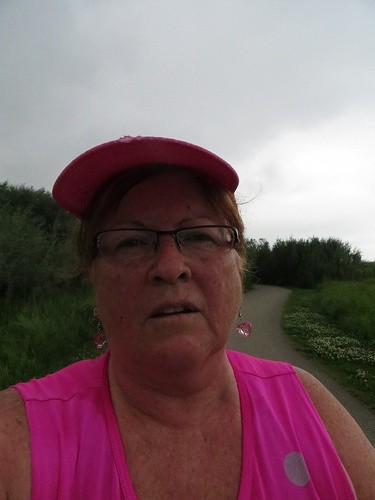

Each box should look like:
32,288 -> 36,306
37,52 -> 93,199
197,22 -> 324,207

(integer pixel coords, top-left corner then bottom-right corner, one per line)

92,224 -> 240,266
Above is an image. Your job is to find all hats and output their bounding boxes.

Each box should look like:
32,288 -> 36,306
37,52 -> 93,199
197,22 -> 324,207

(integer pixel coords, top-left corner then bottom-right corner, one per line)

51,135 -> 240,219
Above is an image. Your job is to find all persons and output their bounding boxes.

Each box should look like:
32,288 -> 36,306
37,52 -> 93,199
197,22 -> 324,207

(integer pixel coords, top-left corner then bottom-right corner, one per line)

0,135 -> 375,500
